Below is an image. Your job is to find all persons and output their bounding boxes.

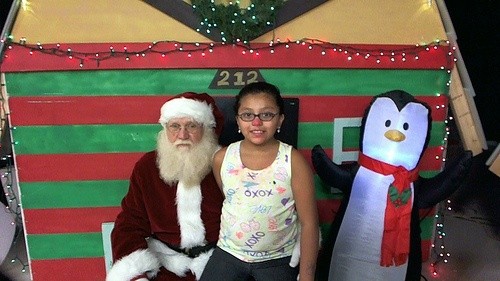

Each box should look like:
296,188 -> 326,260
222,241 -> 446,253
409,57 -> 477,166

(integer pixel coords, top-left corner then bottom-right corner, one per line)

105,92 -> 226,281
198,81 -> 322,281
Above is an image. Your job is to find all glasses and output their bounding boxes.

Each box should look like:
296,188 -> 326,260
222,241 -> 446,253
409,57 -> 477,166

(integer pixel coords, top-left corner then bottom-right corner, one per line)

237,111 -> 281,122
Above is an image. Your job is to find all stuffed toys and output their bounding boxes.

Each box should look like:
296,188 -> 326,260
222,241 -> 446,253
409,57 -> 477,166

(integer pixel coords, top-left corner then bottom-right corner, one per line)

309,90 -> 473,281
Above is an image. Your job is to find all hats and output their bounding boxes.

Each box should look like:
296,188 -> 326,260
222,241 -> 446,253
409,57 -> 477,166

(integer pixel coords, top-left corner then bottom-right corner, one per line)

159,91 -> 225,138
